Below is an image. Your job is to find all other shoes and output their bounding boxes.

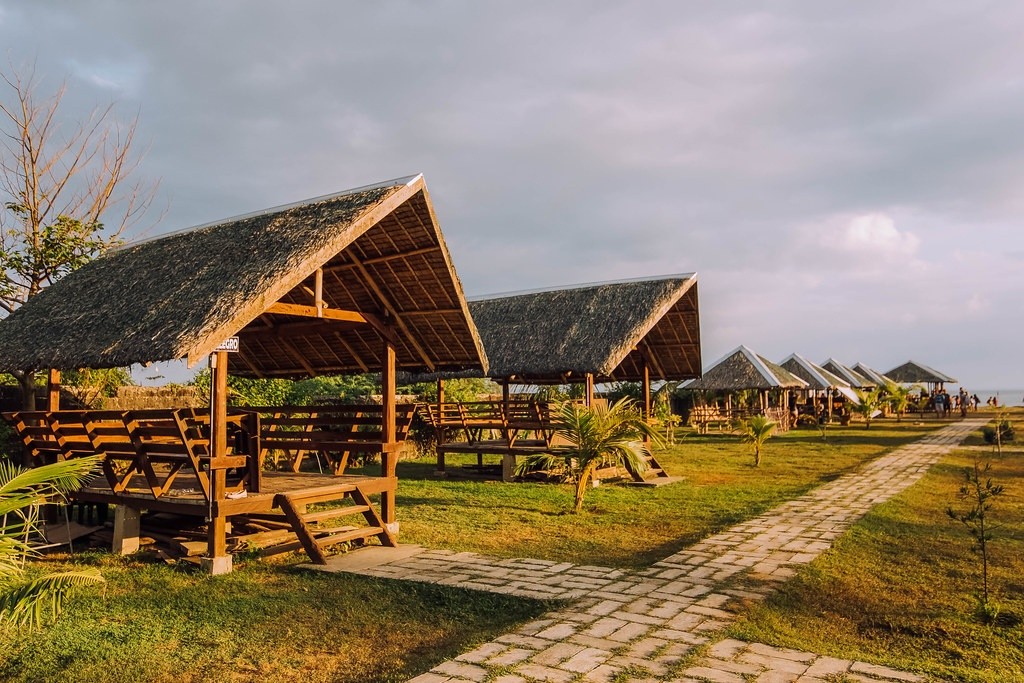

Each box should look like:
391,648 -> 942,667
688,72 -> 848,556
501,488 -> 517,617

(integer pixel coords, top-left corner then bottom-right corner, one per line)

225,488 -> 248,498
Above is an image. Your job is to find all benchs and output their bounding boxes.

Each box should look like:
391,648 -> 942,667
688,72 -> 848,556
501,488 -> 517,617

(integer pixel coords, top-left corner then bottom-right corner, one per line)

691,406 -> 789,431
0,399 -> 564,499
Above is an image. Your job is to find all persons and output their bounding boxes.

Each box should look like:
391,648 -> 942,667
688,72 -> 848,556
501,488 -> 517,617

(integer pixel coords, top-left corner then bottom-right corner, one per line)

788,391 -> 800,428
986,397 -> 998,407
934,387 -> 980,419
